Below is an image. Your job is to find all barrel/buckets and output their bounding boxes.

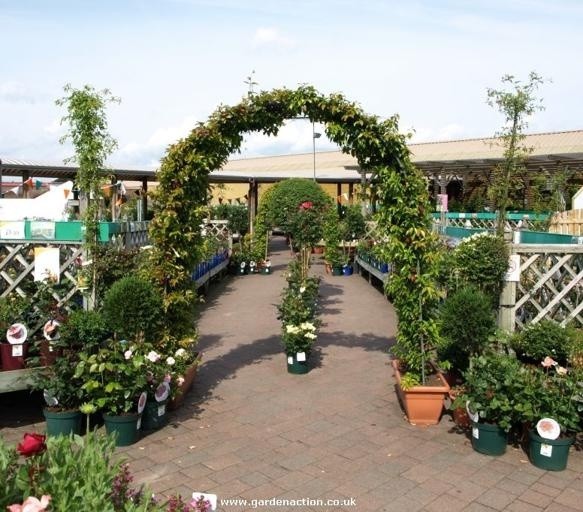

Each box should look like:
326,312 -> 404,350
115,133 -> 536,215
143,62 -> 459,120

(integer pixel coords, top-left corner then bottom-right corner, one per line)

361,254 -> 387,273
43,406 -> 87,439
144,401 -> 168,428
287,348 -> 310,375
527,427 -> 573,472
332,264 -> 351,277
472,420 -> 508,457
236,265 -> 272,276
104,414 -> 142,445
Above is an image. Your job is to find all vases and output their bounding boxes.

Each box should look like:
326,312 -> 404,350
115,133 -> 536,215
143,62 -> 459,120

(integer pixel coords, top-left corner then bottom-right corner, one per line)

288,349 -> 315,374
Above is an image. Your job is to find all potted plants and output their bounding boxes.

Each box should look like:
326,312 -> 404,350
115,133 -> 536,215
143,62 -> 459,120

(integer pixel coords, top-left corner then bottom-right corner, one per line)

36,303 -> 67,369
145,357 -> 173,430
169,338 -> 200,410
230,248 -> 272,276
390,229 -> 453,424
2,297 -> 33,372
527,371 -> 581,472
447,378 -> 470,429
95,355 -> 146,447
322,255 -> 354,277
43,347 -> 84,438
469,369 -> 515,458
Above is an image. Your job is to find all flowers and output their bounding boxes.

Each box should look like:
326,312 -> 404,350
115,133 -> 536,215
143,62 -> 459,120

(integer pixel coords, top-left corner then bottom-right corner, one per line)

285,322 -> 319,347
110,463 -> 215,511
7,495 -> 51,512
17,431 -> 47,465
